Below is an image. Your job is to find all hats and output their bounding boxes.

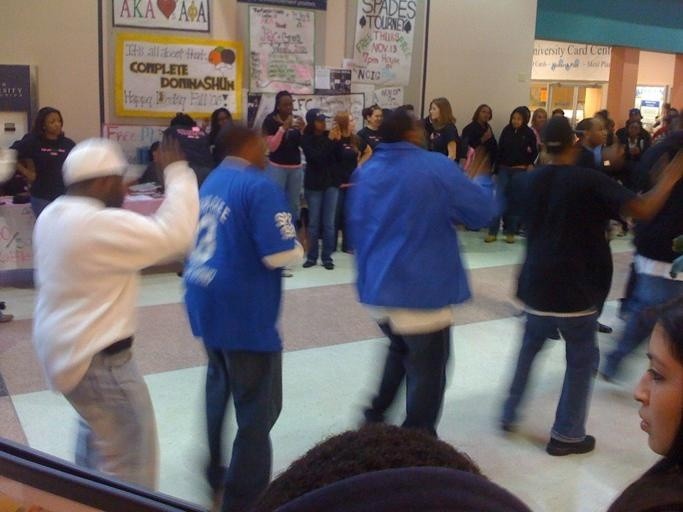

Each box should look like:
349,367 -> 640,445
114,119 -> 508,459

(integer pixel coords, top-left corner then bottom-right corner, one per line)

542,116 -> 582,144
306,109 -> 330,122
61,138 -> 147,186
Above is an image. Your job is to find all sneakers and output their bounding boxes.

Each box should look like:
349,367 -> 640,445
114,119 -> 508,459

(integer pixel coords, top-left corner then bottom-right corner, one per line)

545,426 -> 596,455
303,256 -> 336,269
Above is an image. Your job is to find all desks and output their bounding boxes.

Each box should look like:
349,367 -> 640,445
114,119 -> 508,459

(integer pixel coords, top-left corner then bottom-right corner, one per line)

0,190 -> 165,286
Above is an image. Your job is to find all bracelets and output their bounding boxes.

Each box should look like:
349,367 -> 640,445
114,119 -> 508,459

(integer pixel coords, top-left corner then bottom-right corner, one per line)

279,127 -> 284,132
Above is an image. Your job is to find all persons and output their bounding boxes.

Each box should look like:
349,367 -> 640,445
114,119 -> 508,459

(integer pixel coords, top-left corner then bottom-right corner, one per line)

498,114 -> 682,456
33,135 -> 200,492
607,292 -> 683,510
181,123 -> 310,512
347,104 -> 502,439
146,90 -> 382,271
250,423 -> 531,511
14,107 -> 77,219
421,97 -> 682,244
